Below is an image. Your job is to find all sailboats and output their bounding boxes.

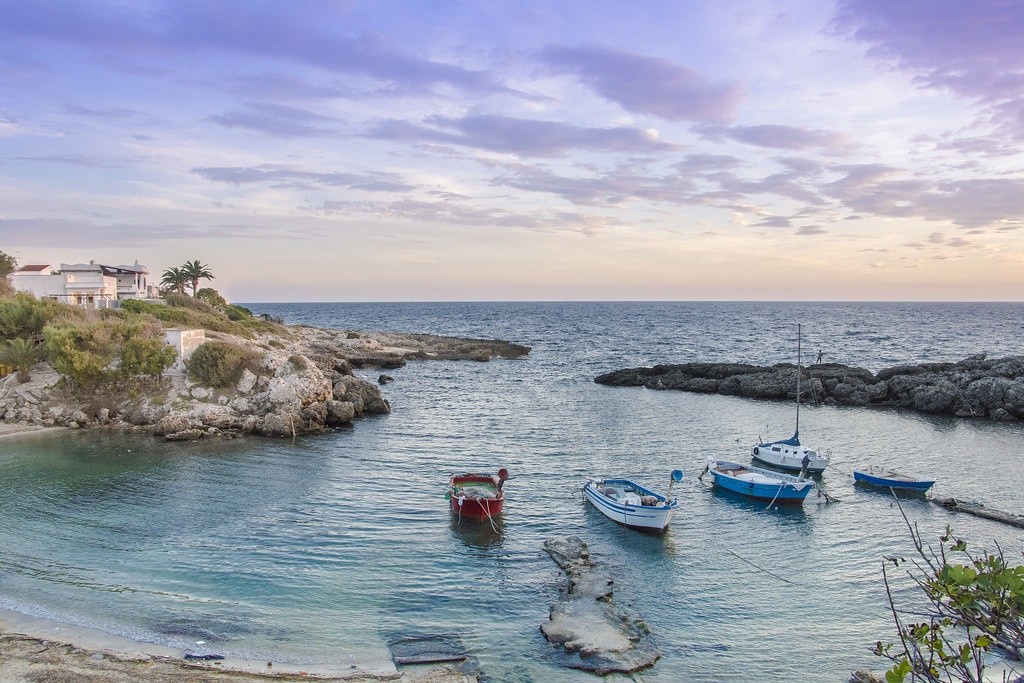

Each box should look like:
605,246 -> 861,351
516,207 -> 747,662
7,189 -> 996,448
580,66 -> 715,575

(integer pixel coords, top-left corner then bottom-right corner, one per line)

750,323 -> 832,476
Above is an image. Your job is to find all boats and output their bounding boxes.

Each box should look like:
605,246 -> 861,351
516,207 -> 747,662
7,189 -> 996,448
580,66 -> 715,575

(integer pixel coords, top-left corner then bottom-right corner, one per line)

853,462 -> 936,494
580,470 -> 683,536
445,468 -> 509,525
698,456 -> 815,503
